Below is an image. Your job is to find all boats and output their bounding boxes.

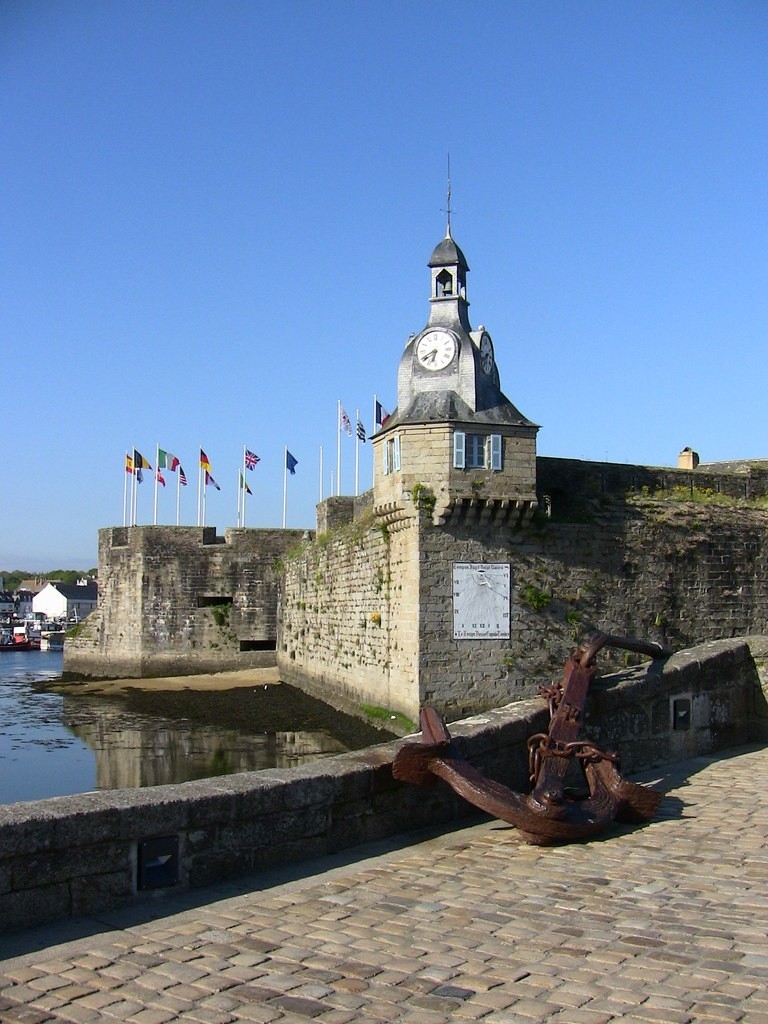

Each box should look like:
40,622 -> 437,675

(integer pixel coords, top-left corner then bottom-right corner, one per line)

0,606 -> 62,651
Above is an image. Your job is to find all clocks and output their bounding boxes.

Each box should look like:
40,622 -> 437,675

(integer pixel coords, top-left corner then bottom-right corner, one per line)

413,326 -> 459,372
480,335 -> 493,375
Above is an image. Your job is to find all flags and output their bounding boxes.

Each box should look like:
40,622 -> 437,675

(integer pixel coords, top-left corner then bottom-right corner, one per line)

356,419 -> 366,443
238,473 -> 253,495
132,449 -> 153,470
284,450 -> 298,475
125,454 -> 144,484
199,449 -> 211,472
204,470 -> 220,490
374,400 -> 392,426
244,449 -> 260,472
156,448 -> 180,472
178,466 -> 187,486
155,467 -> 165,487
337,407 -> 352,438
